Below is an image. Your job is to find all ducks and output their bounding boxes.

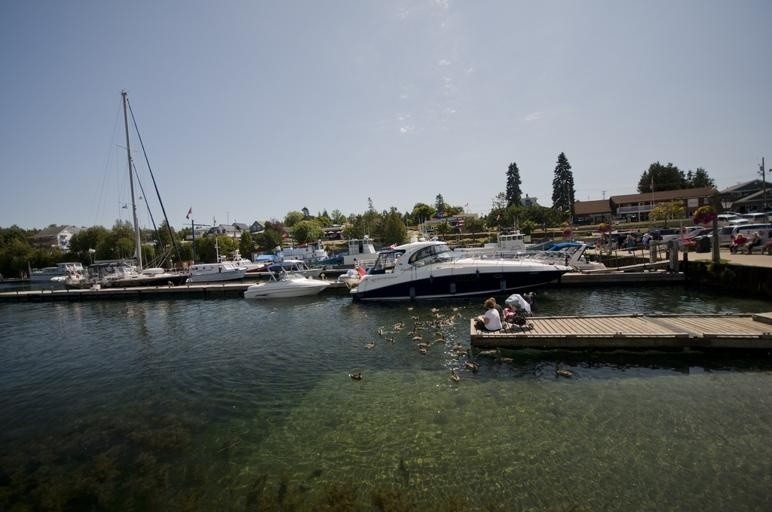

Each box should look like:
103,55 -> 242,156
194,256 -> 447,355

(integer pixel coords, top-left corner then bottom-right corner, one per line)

465,361 -> 481,372
364,306 -> 470,356
554,365 -> 573,377
479,348 -> 501,357
502,357 -> 514,364
449,370 -> 462,382
351,371 -> 363,381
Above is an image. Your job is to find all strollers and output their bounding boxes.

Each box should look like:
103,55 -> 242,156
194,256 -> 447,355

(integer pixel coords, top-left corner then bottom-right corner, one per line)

500,289 -> 537,333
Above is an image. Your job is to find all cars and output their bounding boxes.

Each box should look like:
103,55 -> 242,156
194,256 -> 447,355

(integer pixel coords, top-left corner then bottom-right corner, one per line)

637,214 -> 772,249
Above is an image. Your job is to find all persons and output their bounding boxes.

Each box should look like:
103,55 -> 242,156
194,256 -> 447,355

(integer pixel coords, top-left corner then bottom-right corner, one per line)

498,227 -> 521,240
729,232 -> 762,255
474,299 -> 503,331
490,297 -> 504,323
626,229 -> 654,254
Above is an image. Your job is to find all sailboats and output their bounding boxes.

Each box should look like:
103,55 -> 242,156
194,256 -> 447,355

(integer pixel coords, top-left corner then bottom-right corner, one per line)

65,89 -> 191,290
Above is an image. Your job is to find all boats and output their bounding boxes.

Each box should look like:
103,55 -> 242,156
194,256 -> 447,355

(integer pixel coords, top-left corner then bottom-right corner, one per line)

28,262 -> 84,283
243,265 -> 332,301
349,239 -> 574,304
532,239 -> 609,272
188,239 -> 385,284
